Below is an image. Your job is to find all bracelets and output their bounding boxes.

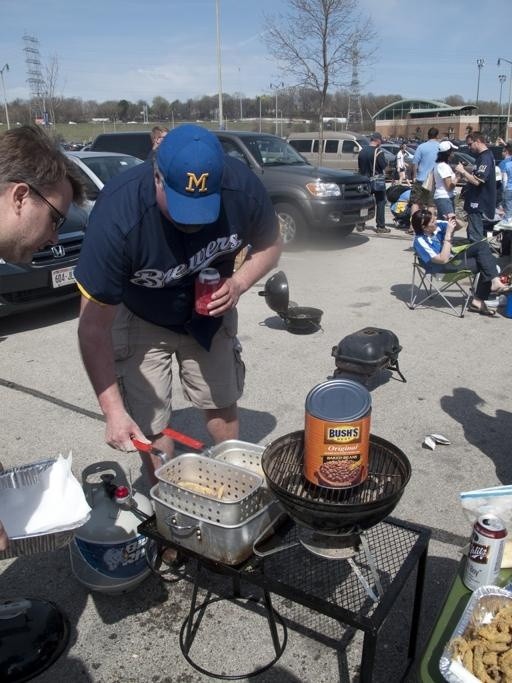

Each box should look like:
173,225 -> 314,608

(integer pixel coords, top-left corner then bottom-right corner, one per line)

442,239 -> 451,243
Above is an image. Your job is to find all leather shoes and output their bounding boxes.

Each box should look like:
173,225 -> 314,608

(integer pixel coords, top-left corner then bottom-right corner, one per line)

161,545 -> 177,565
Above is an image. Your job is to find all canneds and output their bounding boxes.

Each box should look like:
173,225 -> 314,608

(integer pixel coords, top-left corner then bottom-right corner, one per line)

462,515 -> 508,592
303,379 -> 372,489
195,267 -> 222,316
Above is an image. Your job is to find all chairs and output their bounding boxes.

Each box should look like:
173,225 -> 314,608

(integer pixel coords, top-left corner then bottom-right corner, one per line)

409,243 -> 480,318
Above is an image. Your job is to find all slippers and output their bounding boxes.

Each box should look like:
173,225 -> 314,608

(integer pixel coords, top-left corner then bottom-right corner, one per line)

469,305 -> 495,316
489,283 -> 512,296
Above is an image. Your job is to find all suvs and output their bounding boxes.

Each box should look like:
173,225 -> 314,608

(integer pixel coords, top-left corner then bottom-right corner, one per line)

208,130 -> 376,250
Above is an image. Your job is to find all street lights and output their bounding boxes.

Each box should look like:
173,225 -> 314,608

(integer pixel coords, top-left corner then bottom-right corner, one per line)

0,62 -> 11,129
476,57 -> 486,106
498,57 -> 512,142
499,74 -> 506,108
498,74 -> 506,116
476,58 -> 484,108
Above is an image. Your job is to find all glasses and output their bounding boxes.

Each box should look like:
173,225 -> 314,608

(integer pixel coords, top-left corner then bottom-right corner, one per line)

9,179 -> 68,232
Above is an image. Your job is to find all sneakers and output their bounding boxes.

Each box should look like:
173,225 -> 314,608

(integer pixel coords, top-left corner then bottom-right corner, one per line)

377,226 -> 391,233
357,225 -> 364,231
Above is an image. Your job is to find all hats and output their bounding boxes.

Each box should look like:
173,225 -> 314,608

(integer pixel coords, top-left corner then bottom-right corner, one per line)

371,131 -> 384,141
439,140 -> 459,151
154,125 -> 226,226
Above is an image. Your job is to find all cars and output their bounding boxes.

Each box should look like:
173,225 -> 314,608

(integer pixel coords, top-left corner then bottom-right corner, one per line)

382,140 -> 418,162
285,131 -> 369,173
89,133 -> 152,160
63,151 -> 144,213
0,204 -> 89,320
450,147 -> 475,185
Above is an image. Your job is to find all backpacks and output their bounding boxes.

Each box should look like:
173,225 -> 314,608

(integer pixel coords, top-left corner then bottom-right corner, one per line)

386,184 -> 413,203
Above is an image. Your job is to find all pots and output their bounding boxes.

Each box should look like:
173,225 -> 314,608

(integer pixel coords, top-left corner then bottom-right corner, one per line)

331,326 -> 403,377
259,270 -> 323,335
262,429 -> 413,536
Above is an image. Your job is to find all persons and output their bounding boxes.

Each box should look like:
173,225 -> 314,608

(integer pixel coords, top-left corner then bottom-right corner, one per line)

395,145 -> 406,185
411,209 -> 512,315
1,123 -> 84,269
466,123 -> 473,135
146,125 -> 170,161
357,132 -> 392,233
391,143 -> 412,187
482,166 -> 505,238
432,140 -> 462,244
497,136 -> 512,256
389,189 -> 411,230
405,127 -> 441,235
455,131 -> 497,244
72,123 -> 284,566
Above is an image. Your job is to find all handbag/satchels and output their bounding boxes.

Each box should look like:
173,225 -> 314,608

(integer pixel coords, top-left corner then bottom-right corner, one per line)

369,148 -> 386,192
